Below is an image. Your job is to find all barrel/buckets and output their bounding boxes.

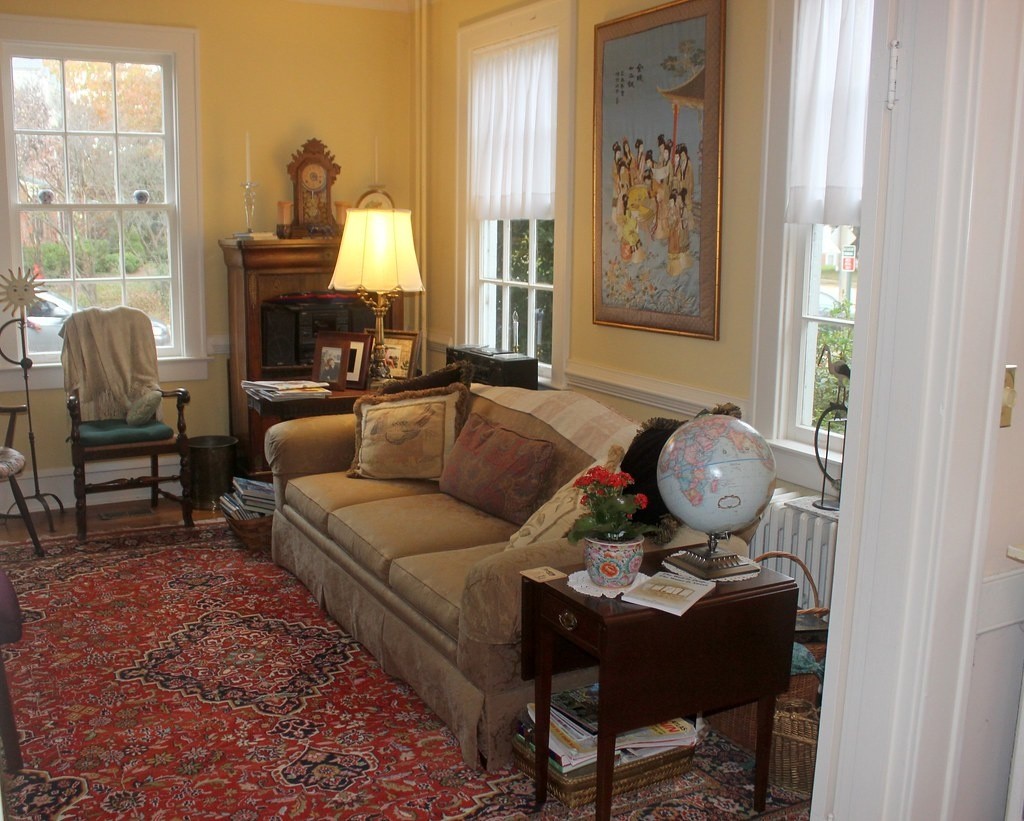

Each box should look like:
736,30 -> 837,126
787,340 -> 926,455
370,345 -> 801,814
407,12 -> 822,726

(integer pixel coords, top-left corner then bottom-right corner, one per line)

187,435 -> 241,511
230,511 -> 272,548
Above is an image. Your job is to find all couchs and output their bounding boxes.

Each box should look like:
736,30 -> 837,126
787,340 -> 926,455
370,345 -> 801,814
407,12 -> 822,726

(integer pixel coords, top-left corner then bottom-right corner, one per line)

264,381 -> 656,775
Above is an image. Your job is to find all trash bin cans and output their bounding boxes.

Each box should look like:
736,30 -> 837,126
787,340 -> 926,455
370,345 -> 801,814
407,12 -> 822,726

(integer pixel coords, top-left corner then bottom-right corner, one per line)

186,436 -> 240,510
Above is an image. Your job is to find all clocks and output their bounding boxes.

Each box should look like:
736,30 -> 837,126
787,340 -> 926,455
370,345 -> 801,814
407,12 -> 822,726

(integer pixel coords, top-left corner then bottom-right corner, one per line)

275,138 -> 341,239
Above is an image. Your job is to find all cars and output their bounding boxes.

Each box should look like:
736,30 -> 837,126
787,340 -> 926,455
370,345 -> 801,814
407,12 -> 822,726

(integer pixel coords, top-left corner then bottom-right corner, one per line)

24,279 -> 172,353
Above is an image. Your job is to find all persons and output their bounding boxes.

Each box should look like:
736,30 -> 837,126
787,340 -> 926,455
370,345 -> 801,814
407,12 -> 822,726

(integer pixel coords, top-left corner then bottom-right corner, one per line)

385,346 -> 395,369
321,358 -> 339,380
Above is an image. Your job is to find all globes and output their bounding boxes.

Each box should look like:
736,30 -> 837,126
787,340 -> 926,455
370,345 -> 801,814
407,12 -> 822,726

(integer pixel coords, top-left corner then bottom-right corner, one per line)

655,415 -> 780,579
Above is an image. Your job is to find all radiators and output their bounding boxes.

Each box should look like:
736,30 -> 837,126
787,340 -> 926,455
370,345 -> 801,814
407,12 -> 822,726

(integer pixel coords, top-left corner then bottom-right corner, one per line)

747,486 -> 838,624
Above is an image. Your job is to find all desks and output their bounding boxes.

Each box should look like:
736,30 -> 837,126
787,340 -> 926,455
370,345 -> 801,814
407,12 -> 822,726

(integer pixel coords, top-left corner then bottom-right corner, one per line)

245,376 -> 434,422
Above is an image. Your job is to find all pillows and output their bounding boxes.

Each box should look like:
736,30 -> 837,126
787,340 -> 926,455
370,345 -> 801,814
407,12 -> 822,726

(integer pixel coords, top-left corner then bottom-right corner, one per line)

612,401 -> 742,546
345,385 -> 473,482
438,412 -> 552,526
505,440 -> 630,555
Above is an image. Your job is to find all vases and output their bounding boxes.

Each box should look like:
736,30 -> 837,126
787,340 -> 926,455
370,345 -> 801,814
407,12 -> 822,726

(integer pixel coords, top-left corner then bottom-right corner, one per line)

580,534 -> 646,589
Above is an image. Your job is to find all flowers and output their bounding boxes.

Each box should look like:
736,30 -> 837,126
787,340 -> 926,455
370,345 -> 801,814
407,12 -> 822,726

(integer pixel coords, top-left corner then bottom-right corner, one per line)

565,466 -> 649,545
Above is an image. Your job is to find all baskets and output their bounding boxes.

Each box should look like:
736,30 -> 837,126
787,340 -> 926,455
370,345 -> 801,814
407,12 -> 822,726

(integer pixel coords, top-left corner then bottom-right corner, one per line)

707,550 -> 833,752
770,698 -> 821,804
510,734 -> 696,808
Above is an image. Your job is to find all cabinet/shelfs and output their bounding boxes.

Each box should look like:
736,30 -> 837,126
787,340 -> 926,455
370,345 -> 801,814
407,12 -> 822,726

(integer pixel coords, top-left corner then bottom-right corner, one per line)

220,234 -> 405,468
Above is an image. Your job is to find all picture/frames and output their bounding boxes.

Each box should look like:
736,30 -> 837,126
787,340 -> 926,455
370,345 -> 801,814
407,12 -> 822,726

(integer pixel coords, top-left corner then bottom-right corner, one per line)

360,326 -> 421,380
312,338 -> 351,390
314,330 -> 373,390
591,2 -> 724,339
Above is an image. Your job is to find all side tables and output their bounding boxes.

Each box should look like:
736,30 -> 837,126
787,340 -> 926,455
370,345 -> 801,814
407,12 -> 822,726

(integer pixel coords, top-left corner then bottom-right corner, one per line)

517,550 -> 800,821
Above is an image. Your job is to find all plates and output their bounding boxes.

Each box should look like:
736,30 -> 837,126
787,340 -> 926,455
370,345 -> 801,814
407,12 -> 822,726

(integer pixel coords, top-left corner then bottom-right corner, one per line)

357,189 -> 395,210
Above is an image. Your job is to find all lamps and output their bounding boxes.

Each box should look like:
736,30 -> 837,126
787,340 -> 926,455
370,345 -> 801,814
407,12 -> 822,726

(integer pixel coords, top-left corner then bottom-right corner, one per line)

330,208 -> 424,386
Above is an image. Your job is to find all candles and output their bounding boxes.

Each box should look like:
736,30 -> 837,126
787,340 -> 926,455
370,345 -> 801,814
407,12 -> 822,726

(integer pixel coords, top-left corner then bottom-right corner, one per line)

246,131 -> 251,186
373,135 -> 379,185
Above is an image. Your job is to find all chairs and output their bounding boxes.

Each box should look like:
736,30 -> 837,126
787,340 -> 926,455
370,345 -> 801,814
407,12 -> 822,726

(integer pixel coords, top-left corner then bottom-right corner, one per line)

59,309 -> 195,543
0,405 -> 44,560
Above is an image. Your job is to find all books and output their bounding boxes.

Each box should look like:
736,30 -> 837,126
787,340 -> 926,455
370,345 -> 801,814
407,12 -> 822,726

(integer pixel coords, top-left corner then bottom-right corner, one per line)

241,380 -> 332,402
219,477 -> 275,520
621,571 -> 716,618
515,682 -> 698,780
